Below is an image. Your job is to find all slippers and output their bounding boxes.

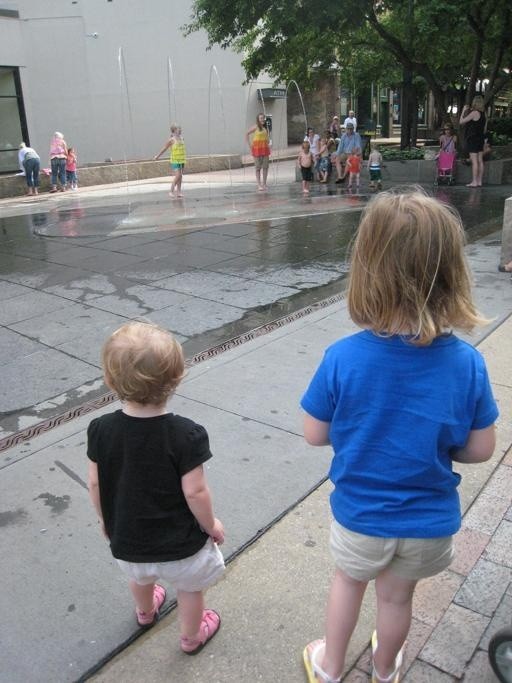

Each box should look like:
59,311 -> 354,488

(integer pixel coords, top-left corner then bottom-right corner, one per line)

303,637 -> 346,683
368,628 -> 408,683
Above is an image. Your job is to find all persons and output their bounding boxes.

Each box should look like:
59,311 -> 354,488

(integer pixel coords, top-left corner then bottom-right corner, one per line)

499,259 -> 512,273
85,322 -> 228,656
463,128 -> 493,166
152,123 -> 187,197
300,185 -> 500,683
437,123 -> 458,183
31,200 -> 86,236
18,131 -> 78,195
459,95 -> 486,188
244,113 -> 271,191
298,110 -> 363,192
367,145 -> 383,187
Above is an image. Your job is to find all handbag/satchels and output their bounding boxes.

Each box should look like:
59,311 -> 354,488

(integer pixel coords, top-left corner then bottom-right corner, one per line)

483,143 -> 491,154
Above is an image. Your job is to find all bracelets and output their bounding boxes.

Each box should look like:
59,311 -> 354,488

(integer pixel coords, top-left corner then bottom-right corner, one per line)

154,156 -> 158,159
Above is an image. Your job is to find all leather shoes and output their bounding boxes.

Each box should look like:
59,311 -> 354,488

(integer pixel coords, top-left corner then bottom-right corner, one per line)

498,264 -> 512,272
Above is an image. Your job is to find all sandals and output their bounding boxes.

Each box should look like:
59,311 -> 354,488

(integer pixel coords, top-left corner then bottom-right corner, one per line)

178,608 -> 221,655
169,192 -> 184,197
26,184 -> 80,196
134,583 -> 166,628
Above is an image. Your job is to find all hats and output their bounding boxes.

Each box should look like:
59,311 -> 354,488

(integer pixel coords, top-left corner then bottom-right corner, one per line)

333,116 -> 339,120
346,123 -> 354,129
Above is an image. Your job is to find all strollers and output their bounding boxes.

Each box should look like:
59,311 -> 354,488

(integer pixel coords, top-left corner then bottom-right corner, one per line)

432,147 -> 456,185
